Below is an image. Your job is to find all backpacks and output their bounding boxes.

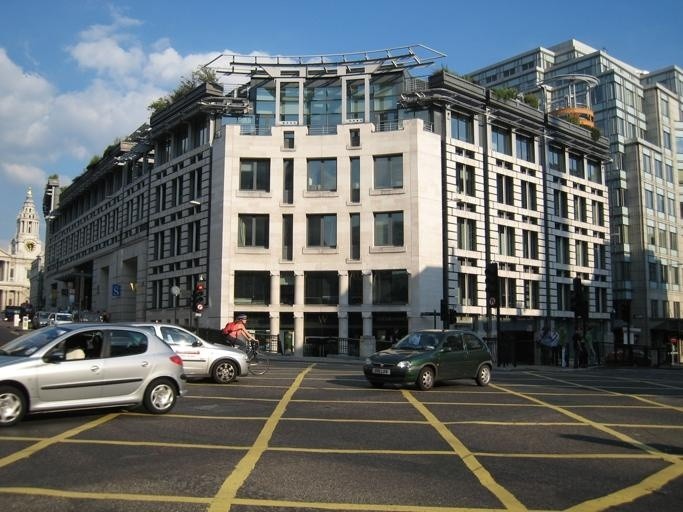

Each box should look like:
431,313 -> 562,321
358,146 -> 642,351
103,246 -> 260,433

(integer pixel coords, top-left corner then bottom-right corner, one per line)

222,322 -> 235,335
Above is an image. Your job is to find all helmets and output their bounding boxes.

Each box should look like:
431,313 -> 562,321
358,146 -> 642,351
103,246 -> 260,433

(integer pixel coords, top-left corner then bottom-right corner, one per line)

237,314 -> 247,319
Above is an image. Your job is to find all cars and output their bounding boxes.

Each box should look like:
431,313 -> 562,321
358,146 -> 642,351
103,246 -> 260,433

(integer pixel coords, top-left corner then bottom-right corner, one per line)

1,311 -> 249,425
364,330 -> 494,390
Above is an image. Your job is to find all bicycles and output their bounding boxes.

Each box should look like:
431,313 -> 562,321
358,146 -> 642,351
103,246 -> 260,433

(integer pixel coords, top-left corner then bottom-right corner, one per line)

247,339 -> 270,375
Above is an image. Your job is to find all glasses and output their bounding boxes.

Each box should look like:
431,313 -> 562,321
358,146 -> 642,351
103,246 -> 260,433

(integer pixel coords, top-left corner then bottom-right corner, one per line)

242,318 -> 248,320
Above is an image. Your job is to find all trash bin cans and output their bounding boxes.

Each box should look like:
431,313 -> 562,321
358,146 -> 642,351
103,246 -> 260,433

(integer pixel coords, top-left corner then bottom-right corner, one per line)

14,314 -> 20,327
284,331 -> 295,355
22,316 -> 28,330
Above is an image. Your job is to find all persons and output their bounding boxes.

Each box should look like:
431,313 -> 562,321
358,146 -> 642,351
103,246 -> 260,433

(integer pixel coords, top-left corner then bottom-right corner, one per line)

68,302 -> 110,323
536,325 -> 597,369
222,314 -> 259,350
390,328 -> 399,346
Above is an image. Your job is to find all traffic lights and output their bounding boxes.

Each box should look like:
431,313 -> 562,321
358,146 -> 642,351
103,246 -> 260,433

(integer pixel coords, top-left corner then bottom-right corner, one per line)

485,267 -> 497,296
195,284 -> 205,304
